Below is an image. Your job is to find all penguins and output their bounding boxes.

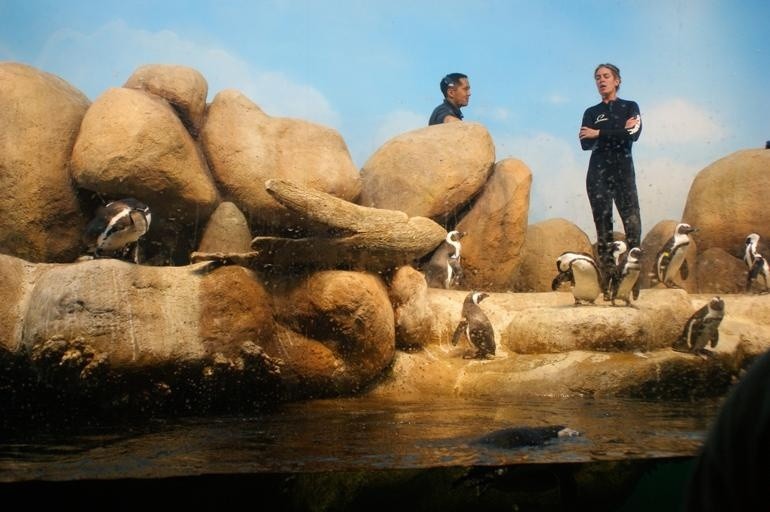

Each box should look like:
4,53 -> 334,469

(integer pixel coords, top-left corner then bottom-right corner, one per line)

452,290 -> 497,361
550,251 -> 608,307
471,422 -> 581,449
428,228 -> 467,288
672,296 -> 725,362
79,197 -> 152,265
605,239 -> 643,307
742,232 -> 770,296
650,222 -> 699,289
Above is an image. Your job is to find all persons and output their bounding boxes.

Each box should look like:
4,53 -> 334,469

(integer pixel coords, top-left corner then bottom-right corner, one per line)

578,62 -> 643,268
428,72 -> 472,127
